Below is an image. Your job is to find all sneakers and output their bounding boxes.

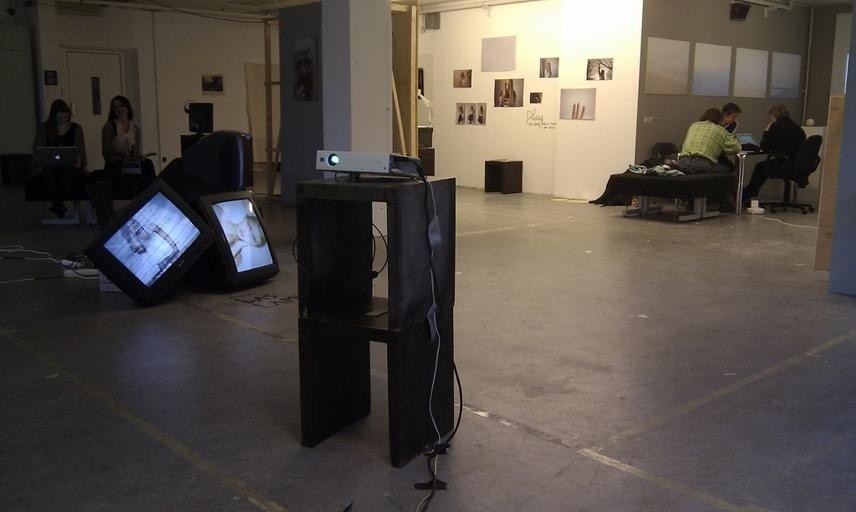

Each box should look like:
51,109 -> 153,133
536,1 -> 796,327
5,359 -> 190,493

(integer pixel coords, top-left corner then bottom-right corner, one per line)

720,202 -> 735,212
50,204 -> 69,217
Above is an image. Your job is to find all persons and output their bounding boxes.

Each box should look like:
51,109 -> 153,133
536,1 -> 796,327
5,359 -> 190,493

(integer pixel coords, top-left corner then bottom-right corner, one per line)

468,106 -> 474,125
718,103 -> 743,173
477,106 -> 484,124
743,103 -> 806,203
498,80 -> 516,108
212,204 -> 266,269
572,103 -> 586,119
678,107 -> 742,212
457,105 -> 465,124
100,95 -> 142,170
31,100 -> 88,219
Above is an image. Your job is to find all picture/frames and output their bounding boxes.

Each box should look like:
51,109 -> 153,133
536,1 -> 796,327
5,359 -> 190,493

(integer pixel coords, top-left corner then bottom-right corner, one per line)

199,74 -> 225,94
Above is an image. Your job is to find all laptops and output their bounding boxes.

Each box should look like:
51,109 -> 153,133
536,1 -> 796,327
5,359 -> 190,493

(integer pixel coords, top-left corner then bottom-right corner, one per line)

35,146 -> 82,169
736,133 -> 763,152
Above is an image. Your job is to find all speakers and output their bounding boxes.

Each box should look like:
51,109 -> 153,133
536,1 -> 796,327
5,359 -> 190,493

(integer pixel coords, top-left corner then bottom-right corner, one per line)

189,103 -> 213,133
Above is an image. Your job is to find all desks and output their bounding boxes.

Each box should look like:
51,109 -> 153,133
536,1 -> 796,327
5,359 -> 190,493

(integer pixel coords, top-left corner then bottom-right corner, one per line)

294,175 -> 457,468
485,160 -> 522,194
732,149 -> 772,214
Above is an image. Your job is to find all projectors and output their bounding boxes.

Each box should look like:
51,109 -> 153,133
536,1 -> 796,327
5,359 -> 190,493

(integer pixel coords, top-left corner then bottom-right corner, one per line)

315,149 -> 421,179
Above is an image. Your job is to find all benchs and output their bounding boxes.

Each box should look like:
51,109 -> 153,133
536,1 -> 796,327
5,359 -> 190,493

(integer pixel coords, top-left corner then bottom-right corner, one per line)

25,176 -> 145,226
607,174 -> 738,221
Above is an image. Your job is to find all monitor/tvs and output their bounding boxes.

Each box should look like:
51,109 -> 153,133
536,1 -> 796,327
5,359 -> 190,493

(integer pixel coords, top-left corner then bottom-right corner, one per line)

184,189 -> 280,293
83,179 -> 218,309
184,130 -> 253,191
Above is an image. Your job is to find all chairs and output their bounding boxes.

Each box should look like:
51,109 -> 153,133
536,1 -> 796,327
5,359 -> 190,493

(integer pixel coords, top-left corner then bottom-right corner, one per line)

763,135 -> 822,214
